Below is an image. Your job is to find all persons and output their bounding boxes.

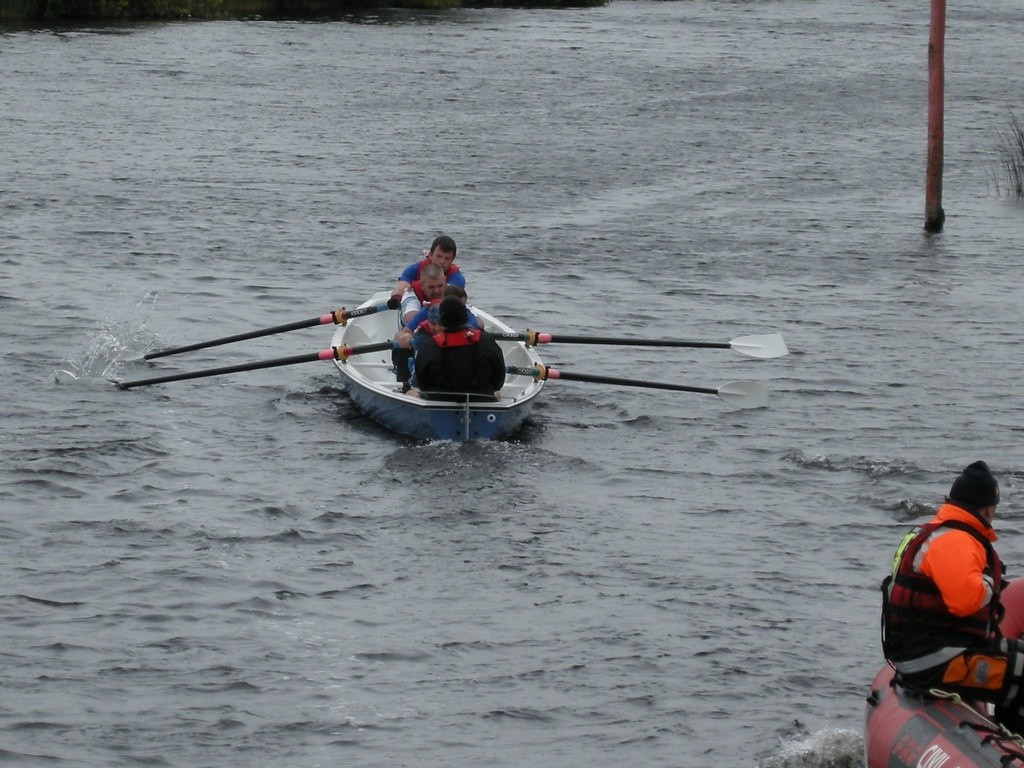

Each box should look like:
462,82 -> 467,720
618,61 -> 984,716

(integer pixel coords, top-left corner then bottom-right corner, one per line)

879,460 -> 1023,736
390,235 -> 508,403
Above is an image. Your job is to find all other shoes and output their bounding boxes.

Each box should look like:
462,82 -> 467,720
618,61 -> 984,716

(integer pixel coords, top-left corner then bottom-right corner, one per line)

402,385 -> 411,393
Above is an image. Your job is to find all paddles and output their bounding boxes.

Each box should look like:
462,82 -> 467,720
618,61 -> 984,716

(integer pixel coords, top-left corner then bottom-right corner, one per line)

51,335 -> 396,393
116,289 -> 402,363
486,332 -> 788,360
503,364 -> 775,410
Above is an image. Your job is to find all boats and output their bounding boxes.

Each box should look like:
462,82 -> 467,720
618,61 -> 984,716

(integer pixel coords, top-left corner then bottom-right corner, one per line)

327,288 -> 548,444
864,658 -> 1024,767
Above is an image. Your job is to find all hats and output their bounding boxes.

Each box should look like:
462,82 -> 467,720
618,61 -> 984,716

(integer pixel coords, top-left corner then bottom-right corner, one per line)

438,295 -> 469,327
949,461 -> 1000,509
